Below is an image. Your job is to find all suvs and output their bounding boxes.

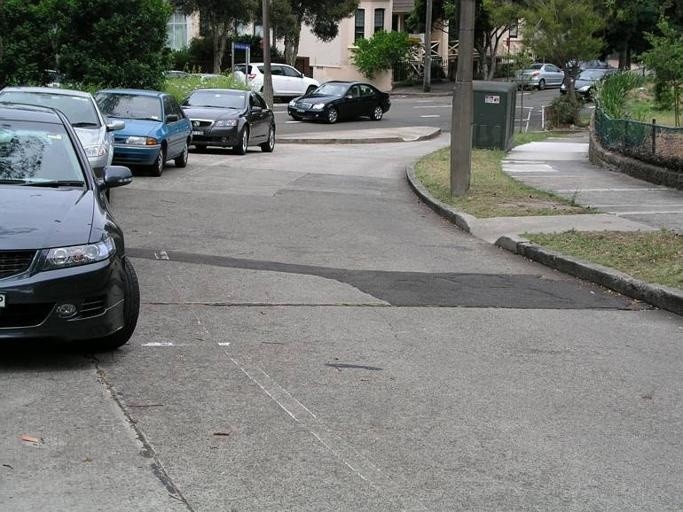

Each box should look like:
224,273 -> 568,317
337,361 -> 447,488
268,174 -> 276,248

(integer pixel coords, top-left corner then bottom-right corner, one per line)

234,62 -> 320,97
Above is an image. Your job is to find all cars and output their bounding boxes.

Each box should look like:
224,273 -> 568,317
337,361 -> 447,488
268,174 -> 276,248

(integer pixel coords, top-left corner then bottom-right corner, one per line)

1,102 -> 140,343
162,71 -> 190,78
287,81 -> 390,121
178,74 -> 229,85
179,89 -> 275,155
94,89 -> 193,176
515,63 -> 564,89
0,84 -> 125,202
560,69 -> 618,101
